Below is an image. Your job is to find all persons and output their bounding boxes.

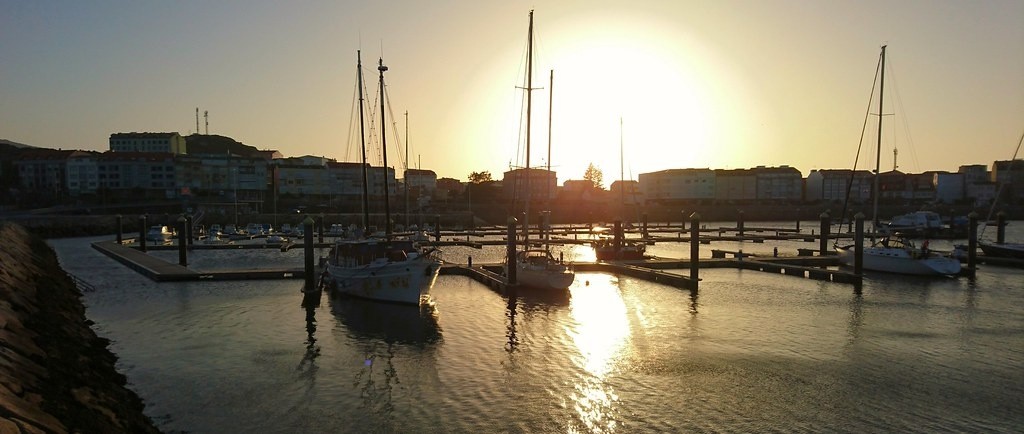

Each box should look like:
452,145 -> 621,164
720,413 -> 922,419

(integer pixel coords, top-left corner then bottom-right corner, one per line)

918,238 -> 932,259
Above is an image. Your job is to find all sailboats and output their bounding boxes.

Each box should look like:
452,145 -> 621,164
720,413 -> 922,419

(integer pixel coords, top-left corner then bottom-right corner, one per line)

832,44 -> 964,276
977,132 -> 1024,259
591,118 -> 650,261
501,8 -> 578,292
325,45 -> 446,307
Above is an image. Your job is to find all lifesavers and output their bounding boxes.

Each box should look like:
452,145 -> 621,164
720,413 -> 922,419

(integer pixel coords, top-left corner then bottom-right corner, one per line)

423,248 -> 430,259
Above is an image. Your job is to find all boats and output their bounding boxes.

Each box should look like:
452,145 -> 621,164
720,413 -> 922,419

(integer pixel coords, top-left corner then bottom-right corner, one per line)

145,169 -> 638,240
875,209 -> 968,241
985,221 -> 1009,227
